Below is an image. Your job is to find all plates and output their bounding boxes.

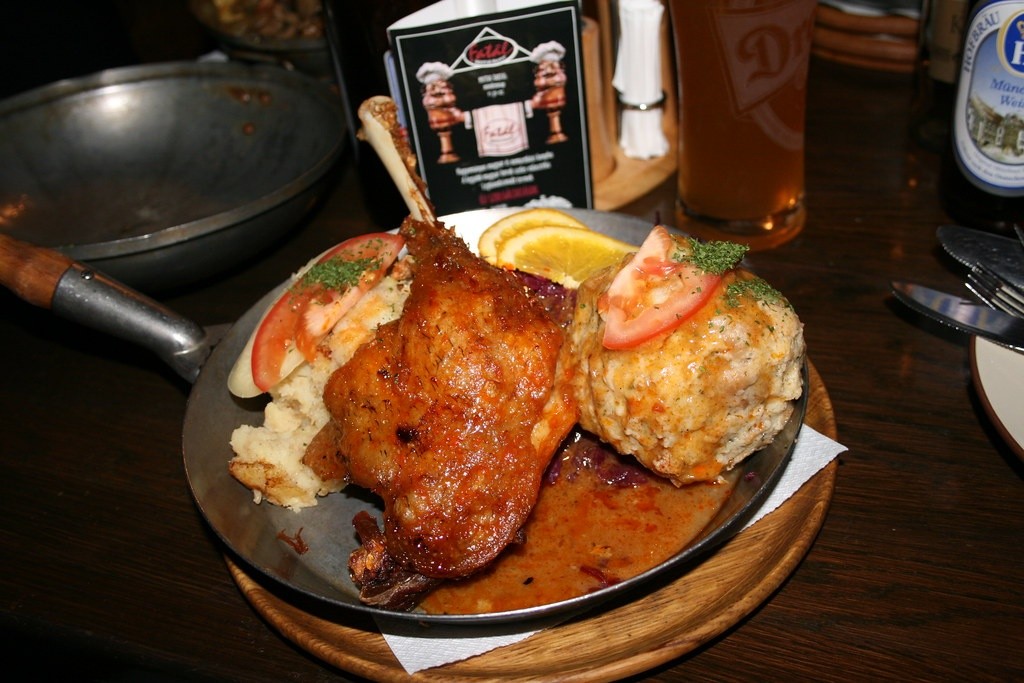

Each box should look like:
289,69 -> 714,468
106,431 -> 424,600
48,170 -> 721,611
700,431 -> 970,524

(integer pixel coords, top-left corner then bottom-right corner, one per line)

808,6 -> 919,80
968,332 -> 1024,463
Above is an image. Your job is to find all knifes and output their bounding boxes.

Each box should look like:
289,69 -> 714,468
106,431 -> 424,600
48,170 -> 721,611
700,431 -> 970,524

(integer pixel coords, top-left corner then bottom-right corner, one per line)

935,224 -> 1024,288
890,282 -> 1024,350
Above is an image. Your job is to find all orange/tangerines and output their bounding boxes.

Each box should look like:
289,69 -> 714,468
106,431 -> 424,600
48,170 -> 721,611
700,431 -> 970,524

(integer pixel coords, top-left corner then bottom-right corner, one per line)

478,207 -> 640,286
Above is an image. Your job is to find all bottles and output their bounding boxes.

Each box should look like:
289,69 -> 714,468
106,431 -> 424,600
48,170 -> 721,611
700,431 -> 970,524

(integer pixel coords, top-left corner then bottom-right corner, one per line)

911,1 -> 971,155
935,1 -> 1024,235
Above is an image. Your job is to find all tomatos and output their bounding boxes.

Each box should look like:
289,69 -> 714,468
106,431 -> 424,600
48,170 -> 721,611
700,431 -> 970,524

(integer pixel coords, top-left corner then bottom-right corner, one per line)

251,232 -> 405,391
598,226 -> 731,349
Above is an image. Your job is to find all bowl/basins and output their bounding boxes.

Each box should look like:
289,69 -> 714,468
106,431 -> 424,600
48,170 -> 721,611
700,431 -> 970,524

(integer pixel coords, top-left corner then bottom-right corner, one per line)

1,58 -> 348,304
194,0 -> 334,76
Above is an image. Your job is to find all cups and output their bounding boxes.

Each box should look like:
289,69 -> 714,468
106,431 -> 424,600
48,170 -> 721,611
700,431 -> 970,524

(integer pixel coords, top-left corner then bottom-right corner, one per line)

581,17 -> 615,184
667,0 -> 817,251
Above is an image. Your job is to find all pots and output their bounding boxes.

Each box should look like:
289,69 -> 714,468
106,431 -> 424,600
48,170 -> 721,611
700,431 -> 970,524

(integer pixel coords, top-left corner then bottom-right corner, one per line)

0,209 -> 807,630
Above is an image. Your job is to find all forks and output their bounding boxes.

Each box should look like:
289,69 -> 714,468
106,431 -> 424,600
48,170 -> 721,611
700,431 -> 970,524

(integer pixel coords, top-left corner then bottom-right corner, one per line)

965,261 -> 1024,321
